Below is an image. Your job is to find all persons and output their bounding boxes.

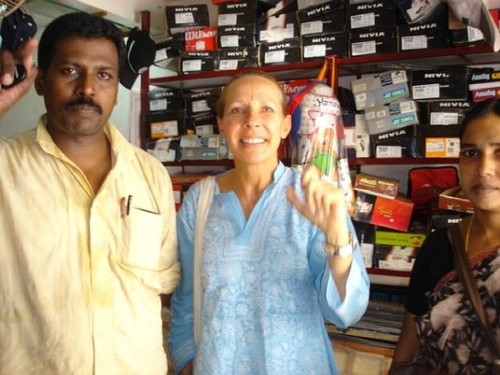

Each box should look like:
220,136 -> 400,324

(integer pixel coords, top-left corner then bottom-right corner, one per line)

170,72 -> 371,374
1,13 -> 181,374
388,98 -> 500,374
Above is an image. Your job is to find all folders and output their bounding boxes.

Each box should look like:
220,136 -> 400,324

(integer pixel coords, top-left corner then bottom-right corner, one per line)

320,296 -> 409,344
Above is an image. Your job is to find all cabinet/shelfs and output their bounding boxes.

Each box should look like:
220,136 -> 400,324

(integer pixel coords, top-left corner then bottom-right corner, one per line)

134,0 -> 499,374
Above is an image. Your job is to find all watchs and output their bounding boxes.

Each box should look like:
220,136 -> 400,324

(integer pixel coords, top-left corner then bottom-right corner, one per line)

323,233 -> 353,256
